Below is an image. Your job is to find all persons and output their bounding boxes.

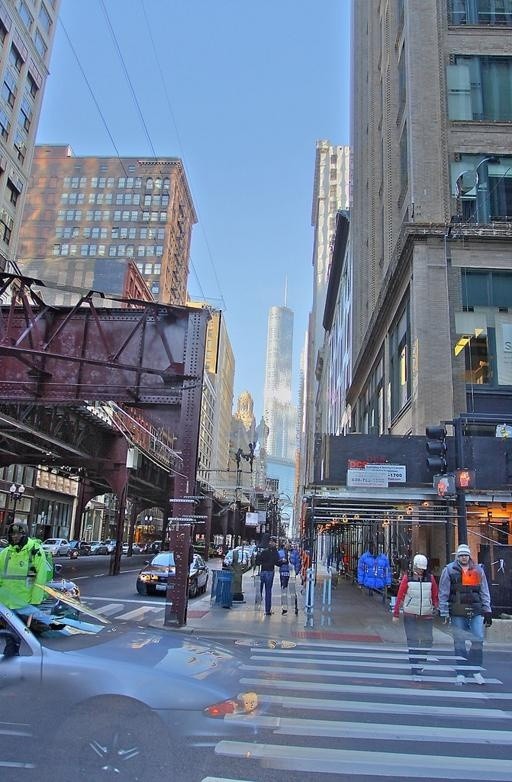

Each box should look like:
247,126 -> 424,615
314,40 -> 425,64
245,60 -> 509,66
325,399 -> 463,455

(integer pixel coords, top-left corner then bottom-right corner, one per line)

252,537 -> 493,688
0,521 -> 53,611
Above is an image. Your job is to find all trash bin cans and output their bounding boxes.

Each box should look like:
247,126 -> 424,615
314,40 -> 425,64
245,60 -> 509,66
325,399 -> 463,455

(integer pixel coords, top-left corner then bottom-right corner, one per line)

70,548 -> 79,559
211,569 -> 233,609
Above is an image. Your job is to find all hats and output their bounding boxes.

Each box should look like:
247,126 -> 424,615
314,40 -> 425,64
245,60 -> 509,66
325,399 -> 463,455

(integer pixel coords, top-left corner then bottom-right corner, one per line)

413,553 -> 428,570
456,543 -> 471,557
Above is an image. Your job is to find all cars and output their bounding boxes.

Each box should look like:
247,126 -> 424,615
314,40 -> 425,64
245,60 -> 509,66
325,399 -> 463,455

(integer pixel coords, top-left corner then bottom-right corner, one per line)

1,580 -> 283,782
194,541 -> 228,559
0,537 -> 163,557
222,546 -> 259,575
135,549 -> 209,599
0,546 -> 83,632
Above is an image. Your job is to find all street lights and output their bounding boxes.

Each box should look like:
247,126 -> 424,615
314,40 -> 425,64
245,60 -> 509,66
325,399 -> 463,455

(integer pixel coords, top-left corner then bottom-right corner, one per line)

8,483 -> 25,544
145,514 -> 152,543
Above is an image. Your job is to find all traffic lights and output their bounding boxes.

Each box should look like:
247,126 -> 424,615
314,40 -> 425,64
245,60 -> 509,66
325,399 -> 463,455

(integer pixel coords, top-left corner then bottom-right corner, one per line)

457,469 -> 478,488
425,425 -> 442,472
434,474 -> 455,499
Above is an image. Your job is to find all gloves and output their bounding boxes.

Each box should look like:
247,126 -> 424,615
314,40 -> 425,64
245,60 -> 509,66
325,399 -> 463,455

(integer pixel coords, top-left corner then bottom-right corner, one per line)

483,612 -> 492,628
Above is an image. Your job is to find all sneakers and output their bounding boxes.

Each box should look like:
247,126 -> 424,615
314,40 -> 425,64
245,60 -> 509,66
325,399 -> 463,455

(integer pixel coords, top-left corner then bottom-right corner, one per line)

281,610 -> 288,616
264,610 -> 275,617
454,673 -> 468,687
411,660 -> 425,682
294,608 -> 299,615
473,672 -> 485,685
